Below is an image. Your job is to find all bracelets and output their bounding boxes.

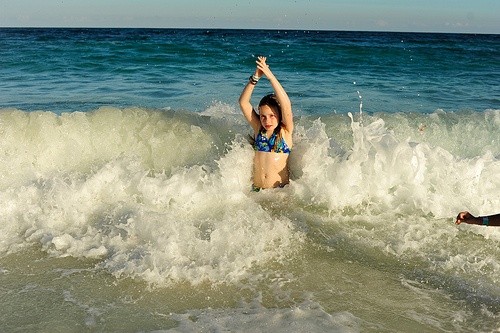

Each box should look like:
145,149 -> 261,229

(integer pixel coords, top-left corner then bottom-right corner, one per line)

482,216 -> 488,226
249,75 -> 260,84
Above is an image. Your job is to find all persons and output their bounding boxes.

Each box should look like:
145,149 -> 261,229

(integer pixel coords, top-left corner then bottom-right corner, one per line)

454,210 -> 500,226
238,55 -> 294,189
